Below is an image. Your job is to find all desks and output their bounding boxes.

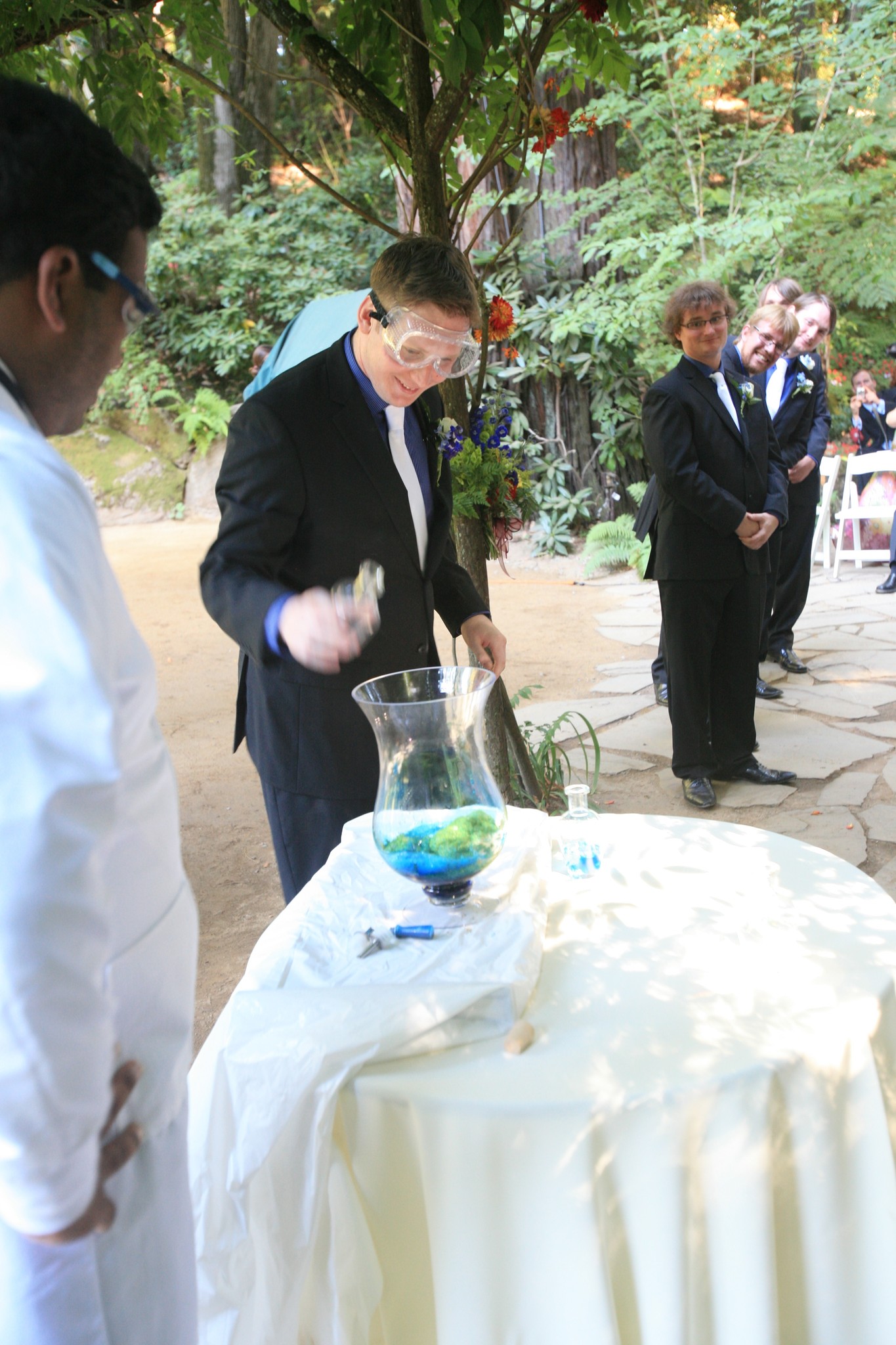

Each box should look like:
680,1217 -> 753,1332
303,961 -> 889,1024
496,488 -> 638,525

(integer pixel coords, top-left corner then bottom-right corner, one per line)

219,818 -> 896,1344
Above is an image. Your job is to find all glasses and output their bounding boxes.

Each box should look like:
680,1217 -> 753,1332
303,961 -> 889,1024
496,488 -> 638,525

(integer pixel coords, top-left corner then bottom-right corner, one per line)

92,252 -> 157,327
369,290 -> 481,379
680,312 -> 728,329
753,325 -> 789,358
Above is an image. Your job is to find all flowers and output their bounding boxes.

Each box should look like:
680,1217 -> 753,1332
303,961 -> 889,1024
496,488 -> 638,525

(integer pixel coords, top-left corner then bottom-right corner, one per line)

473,298 -> 520,361
731,376 -> 763,419
494,75 -> 633,155
790,371 -> 814,399
800,352 -> 816,371
452,385 -> 542,559
434,414 -> 464,483
552,1 -> 623,40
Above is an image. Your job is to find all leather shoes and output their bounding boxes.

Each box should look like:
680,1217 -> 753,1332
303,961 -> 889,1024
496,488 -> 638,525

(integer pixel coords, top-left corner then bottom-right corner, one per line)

716,756 -> 795,785
754,677 -> 783,699
683,777 -> 716,809
876,570 -> 896,592
766,647 -> 807,674
655,682 -> 669,706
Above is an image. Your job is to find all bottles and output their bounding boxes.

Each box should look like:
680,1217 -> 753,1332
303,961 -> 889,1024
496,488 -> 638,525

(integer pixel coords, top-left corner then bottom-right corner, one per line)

550,778 -> 609,888
330,559 -> 381,647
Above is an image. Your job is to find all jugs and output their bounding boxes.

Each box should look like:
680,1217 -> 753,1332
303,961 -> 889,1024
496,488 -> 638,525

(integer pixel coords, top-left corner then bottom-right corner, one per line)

350,660 -> 512,932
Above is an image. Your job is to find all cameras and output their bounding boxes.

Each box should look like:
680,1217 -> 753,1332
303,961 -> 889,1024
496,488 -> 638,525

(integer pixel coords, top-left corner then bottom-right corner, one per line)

857,385 -> 866,401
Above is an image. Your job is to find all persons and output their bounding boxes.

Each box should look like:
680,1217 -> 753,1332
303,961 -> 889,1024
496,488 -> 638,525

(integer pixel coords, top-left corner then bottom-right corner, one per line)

756,282 -> 801,319
2,71 -> 216,1345
847,368 -> 896,499
757,293 -> 838,678
640,276 -> 800,806
875,408 -> 896,595
648,302 -> 800,707
199,237 -> 513,914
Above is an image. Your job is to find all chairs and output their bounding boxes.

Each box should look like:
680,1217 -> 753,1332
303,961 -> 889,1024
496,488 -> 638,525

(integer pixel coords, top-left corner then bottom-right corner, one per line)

806,453 -> 842,570
830,449 -> 896,580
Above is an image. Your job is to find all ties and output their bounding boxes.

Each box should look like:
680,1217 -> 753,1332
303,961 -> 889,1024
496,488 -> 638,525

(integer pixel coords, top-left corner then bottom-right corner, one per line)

872,408 -> 889,450
765,358 -> 788,421
383,403 -> 428,577
710,371 -> 742,434
